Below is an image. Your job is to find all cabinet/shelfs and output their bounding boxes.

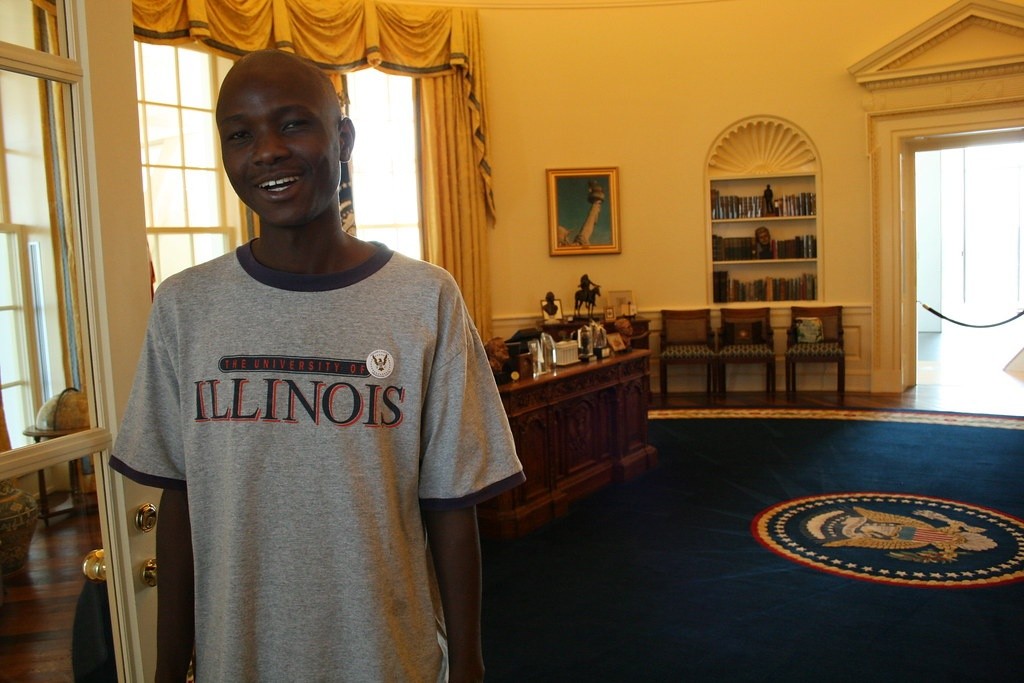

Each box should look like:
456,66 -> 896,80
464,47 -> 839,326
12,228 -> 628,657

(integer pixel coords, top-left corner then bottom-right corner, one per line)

704,172 -> 822,306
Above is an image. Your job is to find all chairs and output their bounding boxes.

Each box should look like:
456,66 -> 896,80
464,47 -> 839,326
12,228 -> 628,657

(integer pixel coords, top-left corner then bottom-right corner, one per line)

716,307 -> 776,399
785,306 -> 845,409
661,307 -> 717,399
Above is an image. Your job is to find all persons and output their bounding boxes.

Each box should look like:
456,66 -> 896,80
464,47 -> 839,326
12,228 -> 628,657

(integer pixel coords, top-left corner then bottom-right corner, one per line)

614,318 -> 633,348
484,337 -> 509,374
578,274 -> 600,309
542,293 -> 559,314
763,184 -> 774,212
755,227 -> 771,257
108,49 -> 526,683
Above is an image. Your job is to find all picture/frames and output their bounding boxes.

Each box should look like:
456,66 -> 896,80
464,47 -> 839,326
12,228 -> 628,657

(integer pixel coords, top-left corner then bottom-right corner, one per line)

605,289 -> 637,317
544,167 -> 623,257
541,299 -> 564,320
603,306 -> 616,320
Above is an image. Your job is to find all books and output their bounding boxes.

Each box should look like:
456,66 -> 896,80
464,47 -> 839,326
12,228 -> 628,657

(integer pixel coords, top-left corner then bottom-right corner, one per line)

582,347 -> 611,364
710,189 -> 816,301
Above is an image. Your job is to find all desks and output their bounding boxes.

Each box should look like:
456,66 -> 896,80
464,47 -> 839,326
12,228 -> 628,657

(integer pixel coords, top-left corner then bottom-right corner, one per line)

22,426 -> 91,526
475,351 -> 656,540
